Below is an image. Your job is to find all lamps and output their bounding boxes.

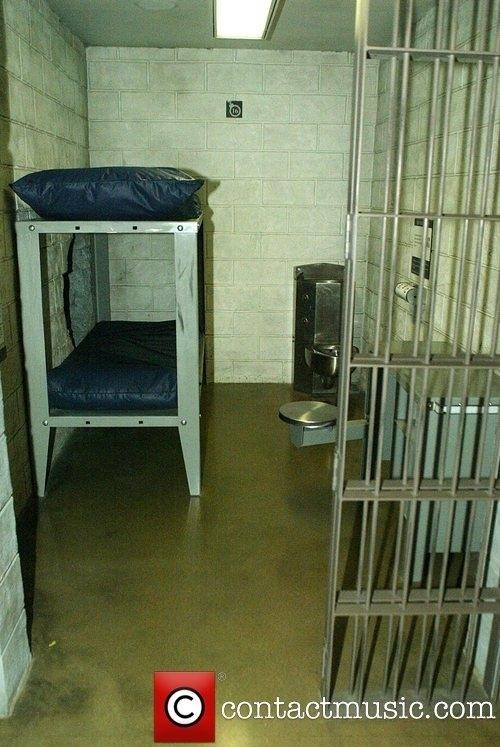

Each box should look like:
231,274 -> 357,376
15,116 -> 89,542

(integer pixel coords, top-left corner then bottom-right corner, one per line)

212,1 -> 286,41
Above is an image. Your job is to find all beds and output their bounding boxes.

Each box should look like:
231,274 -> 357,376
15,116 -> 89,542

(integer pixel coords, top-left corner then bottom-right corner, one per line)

9,168 -> 204,495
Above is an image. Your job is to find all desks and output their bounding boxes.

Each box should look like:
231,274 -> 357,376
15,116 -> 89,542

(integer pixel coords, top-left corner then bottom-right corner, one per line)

367,340 -> 500,553
